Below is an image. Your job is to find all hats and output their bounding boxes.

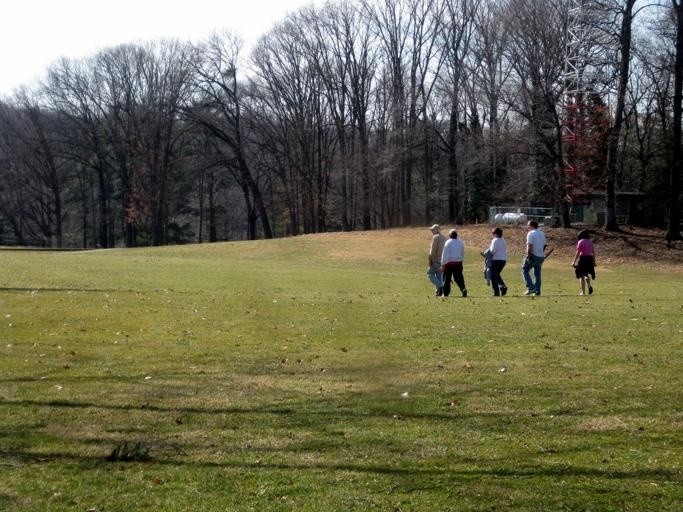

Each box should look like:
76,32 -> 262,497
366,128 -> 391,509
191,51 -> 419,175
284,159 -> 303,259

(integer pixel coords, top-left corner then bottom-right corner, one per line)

428,224 -> 440,231
449,228 -> 458,236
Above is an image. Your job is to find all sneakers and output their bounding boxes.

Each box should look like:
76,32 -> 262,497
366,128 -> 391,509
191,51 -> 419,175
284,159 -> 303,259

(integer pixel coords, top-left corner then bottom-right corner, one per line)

581,286 -> 595,296
435,284 -> 450,296
493,286 -> 508,296
524,286 -> 543,295
462,288 -> 468,297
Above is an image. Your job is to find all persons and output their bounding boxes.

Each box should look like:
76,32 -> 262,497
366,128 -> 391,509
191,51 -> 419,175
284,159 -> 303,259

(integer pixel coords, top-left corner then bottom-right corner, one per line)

521,219 -> 549,295
426,223 -> 467,302
495,207 -> 532,227
481,226 -> 508,298
571,229 -> 599,297
